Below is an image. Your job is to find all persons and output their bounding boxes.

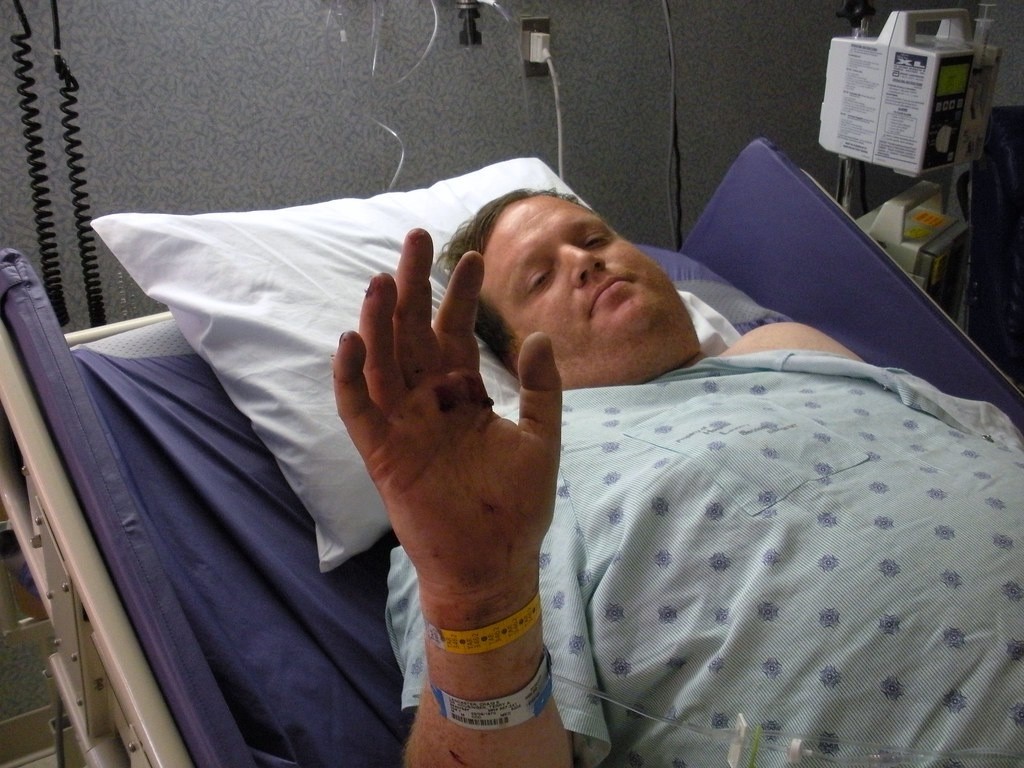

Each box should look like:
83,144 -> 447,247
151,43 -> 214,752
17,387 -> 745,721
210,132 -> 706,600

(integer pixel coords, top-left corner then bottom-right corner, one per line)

333,189 -> 1023,768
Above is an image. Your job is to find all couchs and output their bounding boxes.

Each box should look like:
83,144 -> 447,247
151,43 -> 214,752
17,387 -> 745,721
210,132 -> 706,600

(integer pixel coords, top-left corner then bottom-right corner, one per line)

972,106 -> 1024,388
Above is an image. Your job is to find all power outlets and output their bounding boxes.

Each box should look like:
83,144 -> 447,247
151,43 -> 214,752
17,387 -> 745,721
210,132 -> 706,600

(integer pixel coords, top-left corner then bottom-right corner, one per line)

521,16 -> 549,78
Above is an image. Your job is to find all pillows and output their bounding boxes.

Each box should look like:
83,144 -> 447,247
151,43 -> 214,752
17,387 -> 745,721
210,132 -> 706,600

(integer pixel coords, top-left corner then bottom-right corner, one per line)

89,157 -> 739,572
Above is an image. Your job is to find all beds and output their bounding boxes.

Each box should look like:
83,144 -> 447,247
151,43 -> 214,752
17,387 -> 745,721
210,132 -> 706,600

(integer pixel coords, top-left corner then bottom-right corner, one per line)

0,136 -> 1024,768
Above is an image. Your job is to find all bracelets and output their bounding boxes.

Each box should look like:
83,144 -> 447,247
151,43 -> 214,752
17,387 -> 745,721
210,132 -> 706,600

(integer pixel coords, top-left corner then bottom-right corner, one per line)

423,590 -> 542,655
430,642 -> 552,730
